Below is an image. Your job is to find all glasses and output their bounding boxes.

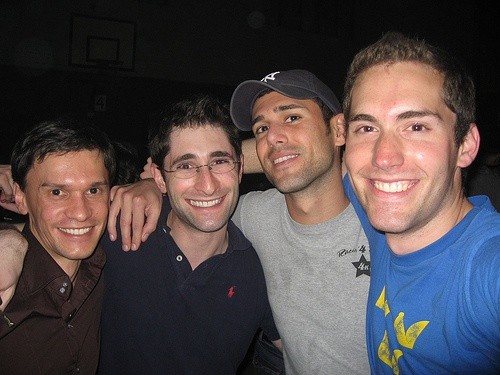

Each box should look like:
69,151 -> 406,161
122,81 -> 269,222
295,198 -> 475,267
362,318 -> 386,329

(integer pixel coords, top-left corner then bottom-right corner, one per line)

158,157 -> 241,179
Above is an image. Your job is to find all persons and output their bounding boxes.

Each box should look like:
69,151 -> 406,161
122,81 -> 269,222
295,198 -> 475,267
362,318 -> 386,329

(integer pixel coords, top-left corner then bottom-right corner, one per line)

0,98 -> 286,375
140,32 -> 500,375
0,116 -> 117,375
107,70 -> 372,375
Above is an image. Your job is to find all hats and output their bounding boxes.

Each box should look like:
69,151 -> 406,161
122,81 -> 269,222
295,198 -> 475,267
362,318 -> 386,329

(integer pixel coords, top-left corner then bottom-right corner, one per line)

230,68 -> 342,131
